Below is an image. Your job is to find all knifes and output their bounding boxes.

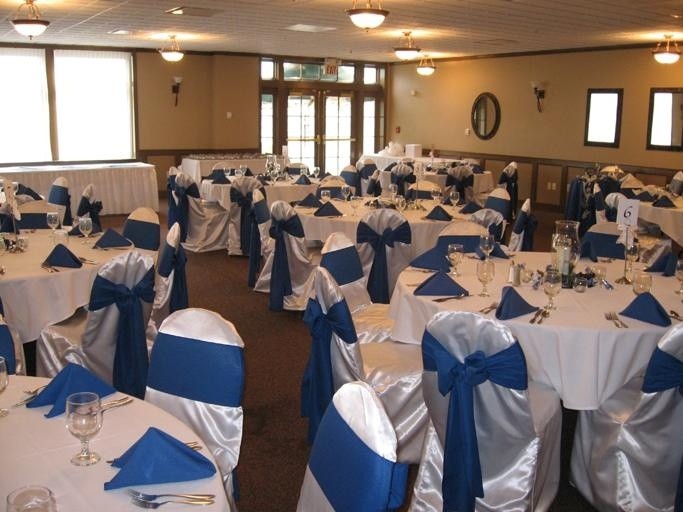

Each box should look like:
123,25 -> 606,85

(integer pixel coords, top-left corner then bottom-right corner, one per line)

11,394 -> 39,408
529,309 -> 543,324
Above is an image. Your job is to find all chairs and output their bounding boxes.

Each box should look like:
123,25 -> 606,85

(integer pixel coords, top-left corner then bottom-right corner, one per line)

467,209 -> 503,245
605,193 -> 627,222
63,183 -> 102,234
173,172 -> 229,253
148,222 -> 188,317
356,208 -> 412,304
212,162 -> 230,175
358,159 -> 377,180
496,161 -> 518,220
47,177 -> 72,227
437,221 -> 489,253
249,189 -> 276,293
143,308 -> 245,512
407,310 -> 562,512
318,180 -> 354,201
405,181 -> 442,201
445,167 -> 474,204
300,266 -> 429,465
316,176 -> 345,197
289,163 -> 310,176
500,198 -> 538,252
319,232 -> 393,344
460,158 -> 483,174
122,207 -> 161,251
167,167 -> 178,230
16,201 -> 62,234
340,166 -> 361,197
390,165 -> 413,196
36,252 -> 156,399
228,177 -> 267,255
581,222 -> 639,261
297,381 -> 397,512
581,181 -> 608,231
484,187 -> 511,219
268,200 -> 323,310
568,324 -> 683,512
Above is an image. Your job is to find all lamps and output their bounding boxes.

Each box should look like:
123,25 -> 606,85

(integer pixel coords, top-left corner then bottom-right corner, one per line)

158,33 -> 185,62
392,31 -> 422,60
651,33 -> 682,64
416,48 -> 436,76
9,0 -> 50,40
345,0 -> 390,32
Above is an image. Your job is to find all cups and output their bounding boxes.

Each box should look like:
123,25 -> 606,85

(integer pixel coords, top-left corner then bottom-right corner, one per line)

47,213 -> 59,239
632,273 -> 650,294
431,187 -> 441,205
234,170 -> 241,177
313,167 -> 320,180
624,243 -> 638,272
477,260 -> 494,297
448,244 -> 462,275
350,196 -> 361,216
300,166 -> 307,176
53,231 -> 67,246
65,393 -> 102,466
395,198 -> 406,215
675,261 -> 683,295
224,167 -> 230,178
0,239 -> 6,275
389,184 -> 398,201
480,235 -> 496,264
271,170 -> 278,184
321,191 -> 329,202
450,192 -> 458,211
544,270 -> 561,310
78,218 -> 93,245
240,165 -> 247,176
0,357 -> 8,418
341,186 -> 350,203
6,485 -> 56,512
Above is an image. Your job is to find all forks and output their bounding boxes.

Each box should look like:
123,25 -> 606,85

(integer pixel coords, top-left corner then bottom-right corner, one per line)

133,499 -> 214,509
604,311 -> 629,328
127,487 -> 212,499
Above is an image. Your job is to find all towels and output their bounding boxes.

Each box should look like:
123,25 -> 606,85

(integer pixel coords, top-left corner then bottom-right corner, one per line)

472,165 -> 484,175
581,239 -> 598,263
94,228 -> 132,251
212,173 -> 231,184
314,200 -> 342,216
444,193 -> 466,205
413,269 -> 469,298
489,241 -> 509,260
25,360 -> 115,416
459,202 -> 482,214
619,188 -> 636,199
652,195 -> 675,208
635,190 -> 654,202
100,424 -> 215,490
297,192 -> 323,208
68,221 -> 103,236
408,247 -> 454,269
294,174 -> 311,186
40,244 -> 81,268
243,167 -> 253,176
425,205 -> 454,222
618,292 -> 672,328
494,285 -> 539,322
644,248 -> 679,277
474,247 -> 485,261
404,188 -> 416,202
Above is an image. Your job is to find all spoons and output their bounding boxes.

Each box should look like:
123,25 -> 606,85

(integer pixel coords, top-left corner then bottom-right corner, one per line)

538,310 -> 550,324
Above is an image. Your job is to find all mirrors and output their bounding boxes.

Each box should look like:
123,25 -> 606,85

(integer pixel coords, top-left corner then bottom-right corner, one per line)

646,87 -> 683,152
471,92 -> 501,140
584,88 -> 623,149
